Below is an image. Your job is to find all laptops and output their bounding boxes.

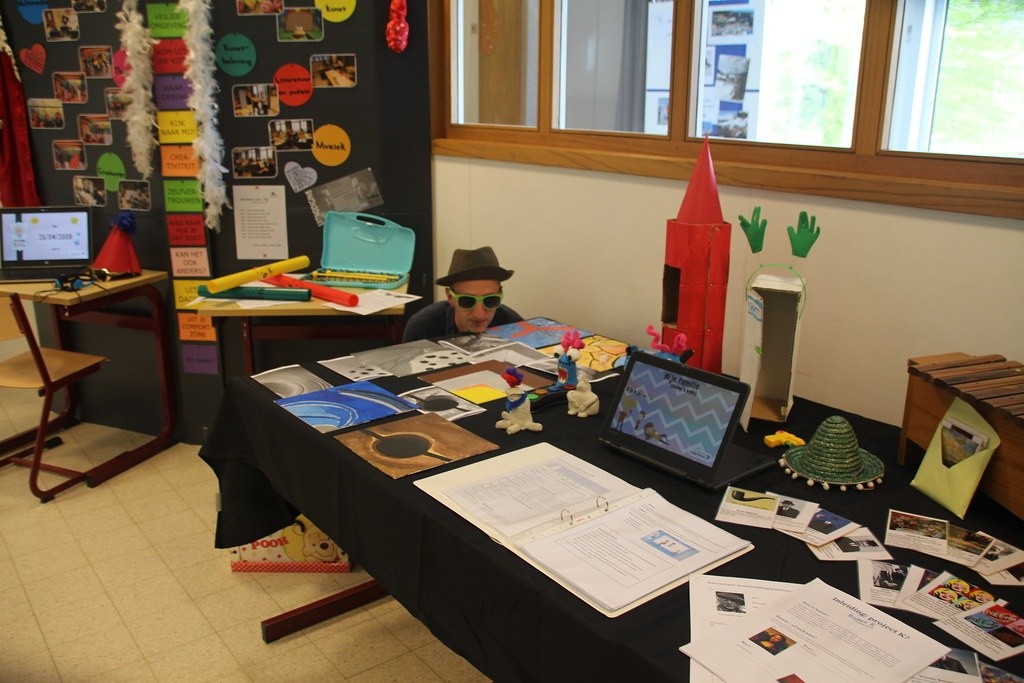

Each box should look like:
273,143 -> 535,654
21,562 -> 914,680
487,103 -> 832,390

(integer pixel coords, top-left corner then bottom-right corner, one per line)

0,206 -> 93,282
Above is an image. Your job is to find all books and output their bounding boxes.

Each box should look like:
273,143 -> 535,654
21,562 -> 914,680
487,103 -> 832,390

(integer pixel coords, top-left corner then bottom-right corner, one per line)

414,441 -> 755,618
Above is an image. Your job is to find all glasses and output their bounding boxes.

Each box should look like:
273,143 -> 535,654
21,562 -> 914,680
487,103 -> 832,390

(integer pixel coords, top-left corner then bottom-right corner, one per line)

449,285 -> 503,310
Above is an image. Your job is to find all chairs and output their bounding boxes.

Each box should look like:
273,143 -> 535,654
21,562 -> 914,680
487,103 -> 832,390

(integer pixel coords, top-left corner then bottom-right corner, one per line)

0,291 -> 113,503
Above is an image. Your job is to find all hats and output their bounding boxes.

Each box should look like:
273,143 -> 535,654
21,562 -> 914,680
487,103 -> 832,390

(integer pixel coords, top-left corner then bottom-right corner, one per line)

779,415 -> 884,488
435,247 -> 514,285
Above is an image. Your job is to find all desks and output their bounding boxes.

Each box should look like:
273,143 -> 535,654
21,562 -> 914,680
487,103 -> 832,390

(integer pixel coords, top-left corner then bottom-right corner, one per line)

197,273 -> 410,375
196,318 -> 1024,683
0,269 -> 182,489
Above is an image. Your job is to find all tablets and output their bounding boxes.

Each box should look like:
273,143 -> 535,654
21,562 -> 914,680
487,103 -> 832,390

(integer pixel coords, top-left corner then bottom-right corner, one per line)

598,350 -> 752,490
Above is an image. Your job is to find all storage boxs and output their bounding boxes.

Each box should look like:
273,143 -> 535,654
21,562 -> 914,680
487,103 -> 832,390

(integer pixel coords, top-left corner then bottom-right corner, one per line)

738,272 -> 807,433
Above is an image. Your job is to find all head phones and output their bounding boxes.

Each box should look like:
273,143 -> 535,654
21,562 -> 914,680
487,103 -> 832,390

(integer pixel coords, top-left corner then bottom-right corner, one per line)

55,273 -> 94,291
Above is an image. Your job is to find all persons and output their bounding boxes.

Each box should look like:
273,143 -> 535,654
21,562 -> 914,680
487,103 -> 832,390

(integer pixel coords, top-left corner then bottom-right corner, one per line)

262,0 -> 283,13
235,157 -> 276,176
716,592 -> 746,613
273,127 -> 305,146
834,536 -> 879,553
113,100 -> 125,117
878,563 -> 908,591
402,246 -> 524,345
931,577 -> 994,610
983,544 -> 1004,561
890,517 -> 943,539
250,91 -> 269,115
46,14 -> 72,38
59,83 -> 84,103
319,56 -> 344,71
87,134 -> 105,144
91,53 -> 110,77
776,500 -> 799,519
56,150 -> 72,167
31,111 -> 63,128
